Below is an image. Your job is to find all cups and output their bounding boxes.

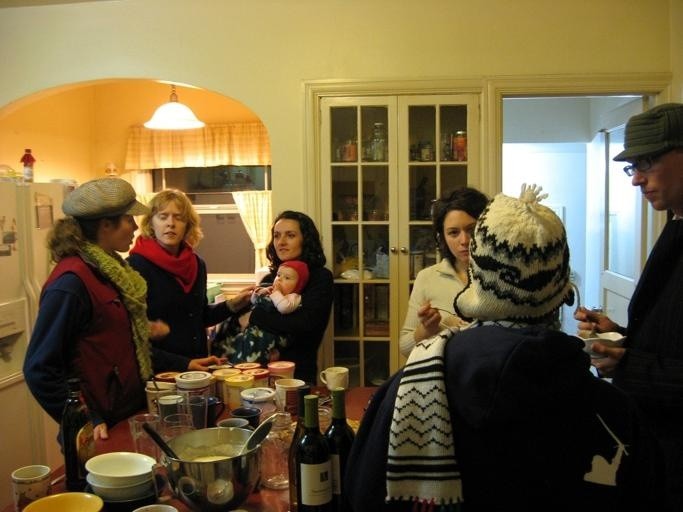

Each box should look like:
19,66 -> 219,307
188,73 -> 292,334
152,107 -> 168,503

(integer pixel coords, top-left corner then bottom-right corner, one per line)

145,381 -> 177,416
176,388 -> 209,429
174,371 -> 215,414
234,362 -> 261,372
187,394 -> 225,430
11,464 -> 50,512
211,368 -> 240,402
274,378 -> 311,415
206,363 -> 233,376
319,366 -> 348,391
153,371 -> 180,383
131,504 -> 178,512
240,368 -> 270,389
154,390 -> 186,425
162,413 -> 192,437
240,387 -> 277,422
127,413 -> 161,461
216,418 -> 254,429
231,406 -> 261,434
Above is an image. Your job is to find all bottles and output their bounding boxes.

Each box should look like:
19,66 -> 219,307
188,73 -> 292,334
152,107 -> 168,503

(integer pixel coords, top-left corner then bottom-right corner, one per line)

242,368 -> 271,387
454,131 -> 468,161
421,144 -> 432,161
62,377 -> 95,488
235,363 -> 261,372
240,387 -> 275,410
260,412 -> 296,490
155,372 -> 180,382
213,369 -> 242,401
409,251 -> 423,280
369,123 -> 387,162
342,140 -> 357,161
207,363 -> 232,370
323,387 -> 356,510
177,371 -> 212,402
20,149 -> 36,183
296,394 -> 332,511
288,385 -> 312,512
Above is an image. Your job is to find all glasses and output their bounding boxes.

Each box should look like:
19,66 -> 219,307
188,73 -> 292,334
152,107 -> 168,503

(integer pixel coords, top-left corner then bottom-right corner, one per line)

623,145 -> 677,176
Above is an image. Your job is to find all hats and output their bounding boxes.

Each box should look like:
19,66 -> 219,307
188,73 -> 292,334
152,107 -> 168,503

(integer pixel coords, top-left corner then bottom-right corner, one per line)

612,102 -> 683,161
281,260 -> 308,294
61,178 -> 150,221
452,189 -> 607,323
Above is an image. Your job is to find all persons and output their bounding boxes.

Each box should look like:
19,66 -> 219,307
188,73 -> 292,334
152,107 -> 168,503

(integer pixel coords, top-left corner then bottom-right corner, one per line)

22,177 -> 153,492
125,189 -> 257,374
221,210 -> 333,392
575,102 -> 683,507
345,193 -> 653,508
399,186 -> 487,358
211,261 -> 308,367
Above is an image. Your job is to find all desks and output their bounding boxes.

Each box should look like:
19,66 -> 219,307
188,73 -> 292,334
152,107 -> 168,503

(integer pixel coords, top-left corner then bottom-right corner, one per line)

0,386 -> 382,512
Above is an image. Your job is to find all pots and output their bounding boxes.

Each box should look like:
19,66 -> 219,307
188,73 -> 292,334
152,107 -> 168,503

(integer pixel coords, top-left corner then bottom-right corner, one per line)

151,426 -> 274,511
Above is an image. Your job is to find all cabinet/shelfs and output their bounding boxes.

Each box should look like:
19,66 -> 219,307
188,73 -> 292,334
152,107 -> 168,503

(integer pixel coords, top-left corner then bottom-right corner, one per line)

0,179 -> 74,512
314,89 -> 481,387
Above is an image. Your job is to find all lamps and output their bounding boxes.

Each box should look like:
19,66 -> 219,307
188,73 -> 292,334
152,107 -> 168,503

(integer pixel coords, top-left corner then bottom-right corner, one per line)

144,86 -> 206,130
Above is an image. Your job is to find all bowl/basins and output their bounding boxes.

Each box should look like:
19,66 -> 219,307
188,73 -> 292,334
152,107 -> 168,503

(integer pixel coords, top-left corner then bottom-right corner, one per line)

84,452 -> 158,512
576,331 -> 626,357
21,491 -> 104,512
